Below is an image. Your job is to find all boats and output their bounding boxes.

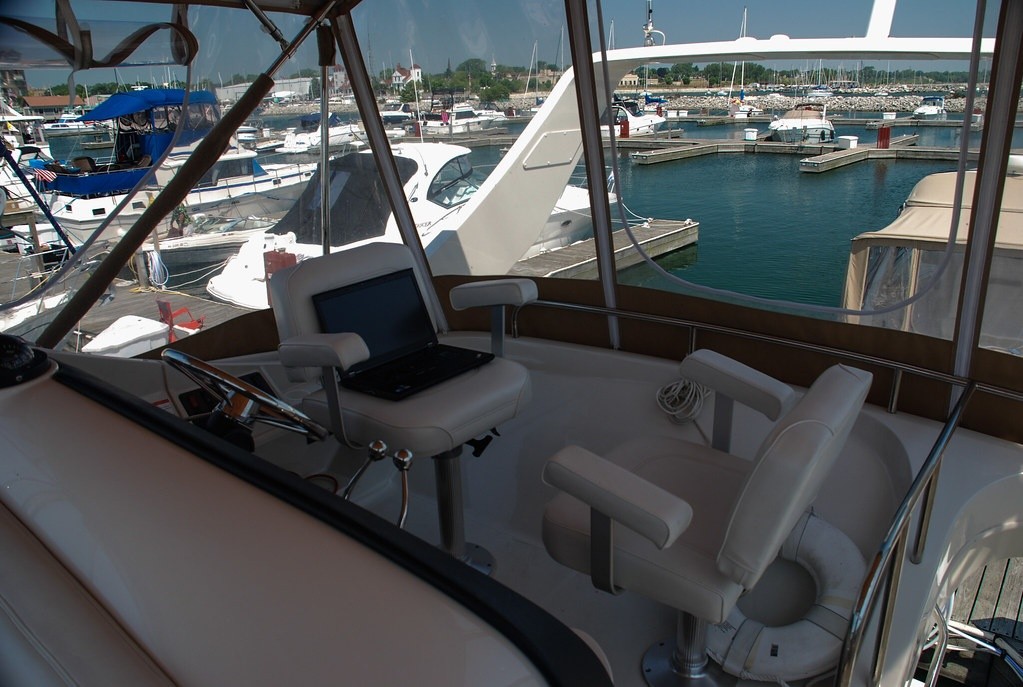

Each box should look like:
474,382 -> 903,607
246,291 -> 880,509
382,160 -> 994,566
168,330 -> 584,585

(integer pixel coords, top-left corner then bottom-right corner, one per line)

766,86 -> 836,144
911,95 -> 948,120
0,24 -> 572,271
599,96 -> 670,139
202,46 -> 625,313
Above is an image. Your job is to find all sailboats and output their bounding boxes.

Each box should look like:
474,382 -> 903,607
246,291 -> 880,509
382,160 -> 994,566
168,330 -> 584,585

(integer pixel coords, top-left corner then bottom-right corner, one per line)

724,4 -> 764,119
632,0 -> 690,116
745,58 -> 990,98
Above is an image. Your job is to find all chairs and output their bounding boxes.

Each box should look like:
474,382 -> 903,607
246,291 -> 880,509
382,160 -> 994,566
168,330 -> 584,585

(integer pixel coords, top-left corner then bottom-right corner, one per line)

269,241 -> 538,575
541,349 -> 873,687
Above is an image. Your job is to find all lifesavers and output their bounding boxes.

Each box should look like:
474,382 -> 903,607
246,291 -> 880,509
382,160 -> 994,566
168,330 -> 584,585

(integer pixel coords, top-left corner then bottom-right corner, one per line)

703,513 -> 868,680
